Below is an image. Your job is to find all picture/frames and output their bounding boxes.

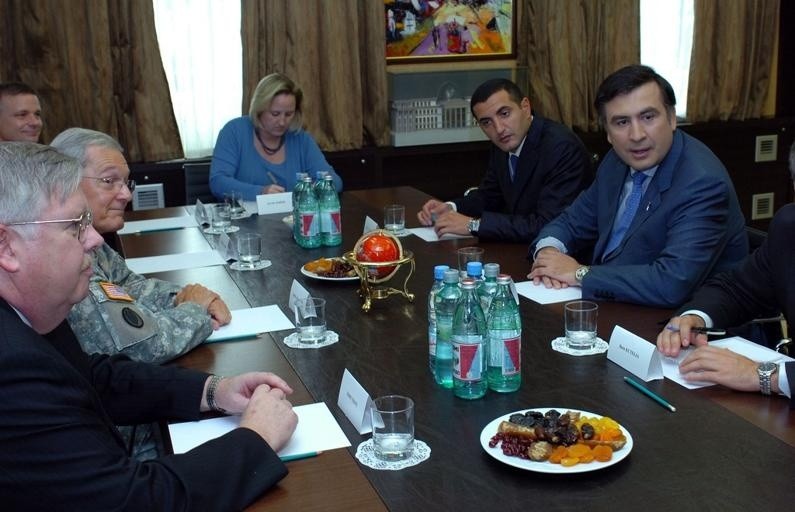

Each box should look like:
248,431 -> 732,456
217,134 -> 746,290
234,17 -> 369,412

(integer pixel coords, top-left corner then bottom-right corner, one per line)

383,0 -> 519,66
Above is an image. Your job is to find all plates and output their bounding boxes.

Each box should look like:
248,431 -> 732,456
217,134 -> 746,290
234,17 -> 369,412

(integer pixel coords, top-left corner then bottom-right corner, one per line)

480,407 -> 634,475
300,257 -> 360,281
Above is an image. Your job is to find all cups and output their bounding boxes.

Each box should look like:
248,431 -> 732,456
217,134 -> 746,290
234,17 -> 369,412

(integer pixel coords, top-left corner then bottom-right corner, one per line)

383,203 -> 405,235
237,232 -> 262,268
212,203 -> 232,232
370,394 -> 414,462
222,190 -> 243,218
564,302 -> 599,350
294,298 -> 326,345
458,247 -> 484,282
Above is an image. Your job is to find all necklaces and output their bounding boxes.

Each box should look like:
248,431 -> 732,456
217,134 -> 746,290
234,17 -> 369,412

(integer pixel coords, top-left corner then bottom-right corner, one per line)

255,126 -> 283,156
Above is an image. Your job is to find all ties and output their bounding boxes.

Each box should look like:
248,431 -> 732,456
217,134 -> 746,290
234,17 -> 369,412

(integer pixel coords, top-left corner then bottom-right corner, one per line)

511,154 -> 520,183
601,172 -> 647,264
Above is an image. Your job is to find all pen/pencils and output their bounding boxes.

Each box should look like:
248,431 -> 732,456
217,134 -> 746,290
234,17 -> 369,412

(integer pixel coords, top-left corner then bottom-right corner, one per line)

625,376 -> 676,413
432,213 -> 438,223
269,172 -> 281,186
204,332 -> 263,344
136,227 -> 185,232
279,452 -> 322,461
668,326 -> 728,335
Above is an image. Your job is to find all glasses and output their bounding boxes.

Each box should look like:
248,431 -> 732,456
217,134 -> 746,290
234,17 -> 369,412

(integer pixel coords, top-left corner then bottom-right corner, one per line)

81,176 -> 136,193
6,209 -> 92,231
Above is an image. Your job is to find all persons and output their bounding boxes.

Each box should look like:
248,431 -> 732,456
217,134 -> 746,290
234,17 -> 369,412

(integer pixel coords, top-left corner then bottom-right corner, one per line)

0,81 -> 43,142
0,139 -> 298,512
49,126 -> 232,460
417,77 -> 595,245
656,139 -> 794,407
207,72 -> 344,202
525,62 -> 750,309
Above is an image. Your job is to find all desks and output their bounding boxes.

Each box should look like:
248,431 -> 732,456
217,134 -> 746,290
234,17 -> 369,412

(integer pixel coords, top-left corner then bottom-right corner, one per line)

98,181 -> 794,512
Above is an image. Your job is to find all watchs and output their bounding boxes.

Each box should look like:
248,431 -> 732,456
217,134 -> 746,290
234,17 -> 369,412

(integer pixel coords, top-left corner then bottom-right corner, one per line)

575,265 -> 590,288
757,362 -> 778,396
467,216 -> 479,234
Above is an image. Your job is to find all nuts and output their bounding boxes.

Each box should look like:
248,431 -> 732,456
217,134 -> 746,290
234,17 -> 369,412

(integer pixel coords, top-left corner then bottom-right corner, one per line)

304,258 -> 356,278
489,410 -> 626,466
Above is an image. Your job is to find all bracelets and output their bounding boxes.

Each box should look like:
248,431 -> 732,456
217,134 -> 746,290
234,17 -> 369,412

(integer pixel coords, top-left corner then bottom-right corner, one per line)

206,375 -> 226,415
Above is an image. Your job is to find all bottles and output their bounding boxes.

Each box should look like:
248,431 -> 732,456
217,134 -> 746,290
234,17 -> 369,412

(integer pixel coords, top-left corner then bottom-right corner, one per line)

427,260 -> 522,400
292,170 -> 343,250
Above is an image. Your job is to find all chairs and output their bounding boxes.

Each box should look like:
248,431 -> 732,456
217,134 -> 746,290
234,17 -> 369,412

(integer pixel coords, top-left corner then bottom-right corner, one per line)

744,225 -> 785,349
183,161 -> 224,205
